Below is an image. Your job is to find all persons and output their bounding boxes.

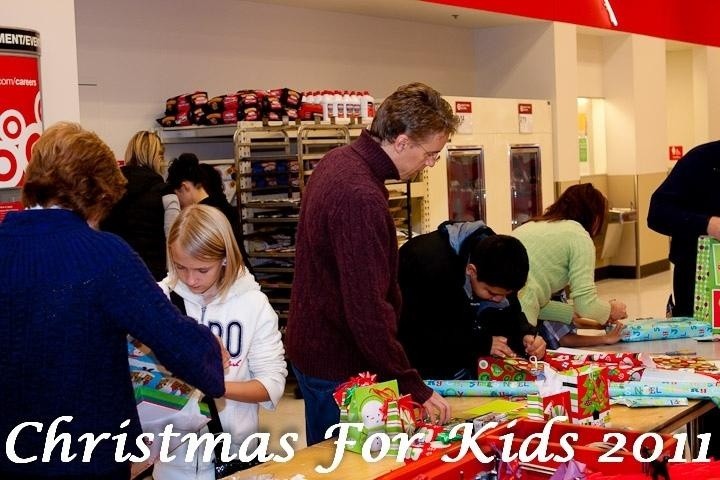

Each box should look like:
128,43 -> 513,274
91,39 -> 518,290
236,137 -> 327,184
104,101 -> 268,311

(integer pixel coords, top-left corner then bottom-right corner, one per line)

509,183 -> 628,336
647,139 -> 720,319
285,81 -> 459,447
538,283 -> 627,349
395,218 -> 547,380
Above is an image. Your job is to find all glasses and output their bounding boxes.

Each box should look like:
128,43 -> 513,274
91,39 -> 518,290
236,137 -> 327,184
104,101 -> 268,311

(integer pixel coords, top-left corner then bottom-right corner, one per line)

418,143 -> 440,161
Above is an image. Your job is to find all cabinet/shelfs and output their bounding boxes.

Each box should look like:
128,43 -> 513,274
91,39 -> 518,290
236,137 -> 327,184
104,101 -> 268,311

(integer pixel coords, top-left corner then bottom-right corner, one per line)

147,114 -> 427,379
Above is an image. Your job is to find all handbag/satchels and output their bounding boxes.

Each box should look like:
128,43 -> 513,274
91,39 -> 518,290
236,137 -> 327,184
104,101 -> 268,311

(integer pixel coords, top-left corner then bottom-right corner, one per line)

694,235 -> 720,333
339,380 -> 415,458
479,357 -> 544,381
528,365 -> 610,425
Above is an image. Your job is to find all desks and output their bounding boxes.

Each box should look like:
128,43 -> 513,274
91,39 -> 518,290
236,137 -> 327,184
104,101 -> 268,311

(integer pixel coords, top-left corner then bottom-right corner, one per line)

212,307 -> 720,480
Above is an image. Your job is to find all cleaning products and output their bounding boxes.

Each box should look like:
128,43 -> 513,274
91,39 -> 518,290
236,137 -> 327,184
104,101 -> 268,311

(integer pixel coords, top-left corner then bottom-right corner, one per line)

301,89 -> 375,125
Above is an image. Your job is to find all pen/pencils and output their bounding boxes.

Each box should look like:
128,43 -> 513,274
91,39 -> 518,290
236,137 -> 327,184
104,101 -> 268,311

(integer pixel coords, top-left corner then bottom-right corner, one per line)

525,331 -> 539,356
562,382 -> 578,388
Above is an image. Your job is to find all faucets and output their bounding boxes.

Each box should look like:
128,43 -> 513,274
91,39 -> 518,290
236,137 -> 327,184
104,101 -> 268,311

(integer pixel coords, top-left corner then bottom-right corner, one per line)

630,201 -> 634,210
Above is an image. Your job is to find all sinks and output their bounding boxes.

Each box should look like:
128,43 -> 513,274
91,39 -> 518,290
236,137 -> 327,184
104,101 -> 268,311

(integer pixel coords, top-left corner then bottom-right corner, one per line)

608,207 -> 635,213
